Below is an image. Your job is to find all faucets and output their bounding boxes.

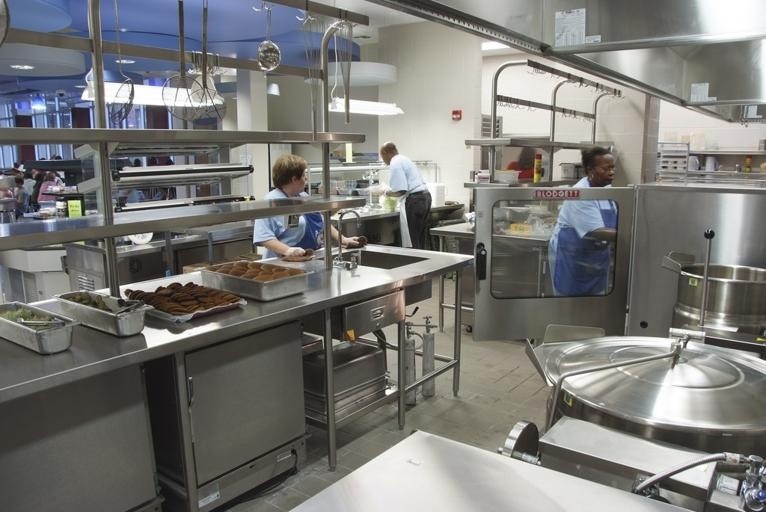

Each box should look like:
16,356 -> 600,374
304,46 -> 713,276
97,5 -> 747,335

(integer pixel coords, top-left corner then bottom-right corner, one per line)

337,210 -> 363,264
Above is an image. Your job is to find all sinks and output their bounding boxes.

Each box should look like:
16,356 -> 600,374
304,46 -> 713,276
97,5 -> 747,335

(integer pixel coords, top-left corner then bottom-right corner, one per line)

316,247 -> 428,269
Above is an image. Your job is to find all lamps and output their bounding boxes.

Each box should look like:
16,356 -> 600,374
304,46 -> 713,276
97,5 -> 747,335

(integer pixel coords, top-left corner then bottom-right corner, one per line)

325,96 -> 404,118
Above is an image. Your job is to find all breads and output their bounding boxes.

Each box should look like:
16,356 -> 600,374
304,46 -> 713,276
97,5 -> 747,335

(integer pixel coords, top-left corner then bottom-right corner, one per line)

204,261 -> 304,281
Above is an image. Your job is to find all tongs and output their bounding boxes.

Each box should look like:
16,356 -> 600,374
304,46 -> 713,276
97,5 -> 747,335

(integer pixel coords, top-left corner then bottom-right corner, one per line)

113,296 -> 145,317
17,315 -> 66,329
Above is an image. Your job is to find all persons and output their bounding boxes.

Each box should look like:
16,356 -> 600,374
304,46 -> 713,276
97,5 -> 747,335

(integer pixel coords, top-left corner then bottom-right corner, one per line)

111,157 -> 178,207
0,151 -> 64,223
378,141 -> 433,251
252,153 -> 369,261
545,143 -> 620,299
501,144 -> 544,185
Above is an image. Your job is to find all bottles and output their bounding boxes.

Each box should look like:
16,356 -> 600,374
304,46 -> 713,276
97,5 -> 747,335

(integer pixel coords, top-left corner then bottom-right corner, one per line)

55,201 -> 68,218
534,152 -> 543,184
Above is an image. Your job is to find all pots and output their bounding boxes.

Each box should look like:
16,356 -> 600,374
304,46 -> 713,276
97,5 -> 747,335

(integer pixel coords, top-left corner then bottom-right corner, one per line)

659,248 -> 766,343
523,319 -> 765,459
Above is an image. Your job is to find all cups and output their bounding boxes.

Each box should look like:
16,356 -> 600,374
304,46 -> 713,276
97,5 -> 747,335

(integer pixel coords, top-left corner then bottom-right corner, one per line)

705,156 -> 720,171
688,155 -> 700,171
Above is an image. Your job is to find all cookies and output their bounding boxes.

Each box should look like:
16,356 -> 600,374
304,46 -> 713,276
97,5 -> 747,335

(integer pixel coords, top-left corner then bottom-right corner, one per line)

124,281 -> 239,316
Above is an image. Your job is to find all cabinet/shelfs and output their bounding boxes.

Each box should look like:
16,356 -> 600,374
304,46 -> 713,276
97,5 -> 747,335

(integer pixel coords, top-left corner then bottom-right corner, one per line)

147,323 -> 310,512
97,40 -> 367,296
465,134 -> 607,188
0,24 -> 115,303
660,143 -> 765,180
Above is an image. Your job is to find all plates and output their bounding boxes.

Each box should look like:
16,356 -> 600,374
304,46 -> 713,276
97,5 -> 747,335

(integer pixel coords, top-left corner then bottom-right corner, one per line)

127,231 -> 153,245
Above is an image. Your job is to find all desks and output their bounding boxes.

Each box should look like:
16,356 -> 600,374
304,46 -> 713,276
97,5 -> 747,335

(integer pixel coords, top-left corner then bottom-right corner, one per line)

427,222 -> 476,333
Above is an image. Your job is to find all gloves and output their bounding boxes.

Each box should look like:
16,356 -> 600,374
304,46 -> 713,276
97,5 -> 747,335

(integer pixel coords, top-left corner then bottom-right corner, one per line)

340,236 -> 360,246
285,247 -> 306,257
